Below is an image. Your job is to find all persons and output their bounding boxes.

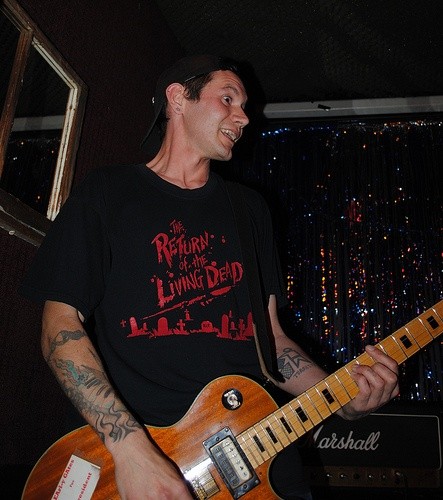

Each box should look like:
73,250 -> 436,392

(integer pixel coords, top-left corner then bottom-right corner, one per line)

19,54 -> 400,500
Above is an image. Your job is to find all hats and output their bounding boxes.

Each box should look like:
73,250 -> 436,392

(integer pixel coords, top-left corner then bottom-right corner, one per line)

140,55 -> 238,146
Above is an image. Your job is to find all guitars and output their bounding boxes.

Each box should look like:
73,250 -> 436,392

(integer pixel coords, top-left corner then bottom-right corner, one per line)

18,298 -> 443,500
